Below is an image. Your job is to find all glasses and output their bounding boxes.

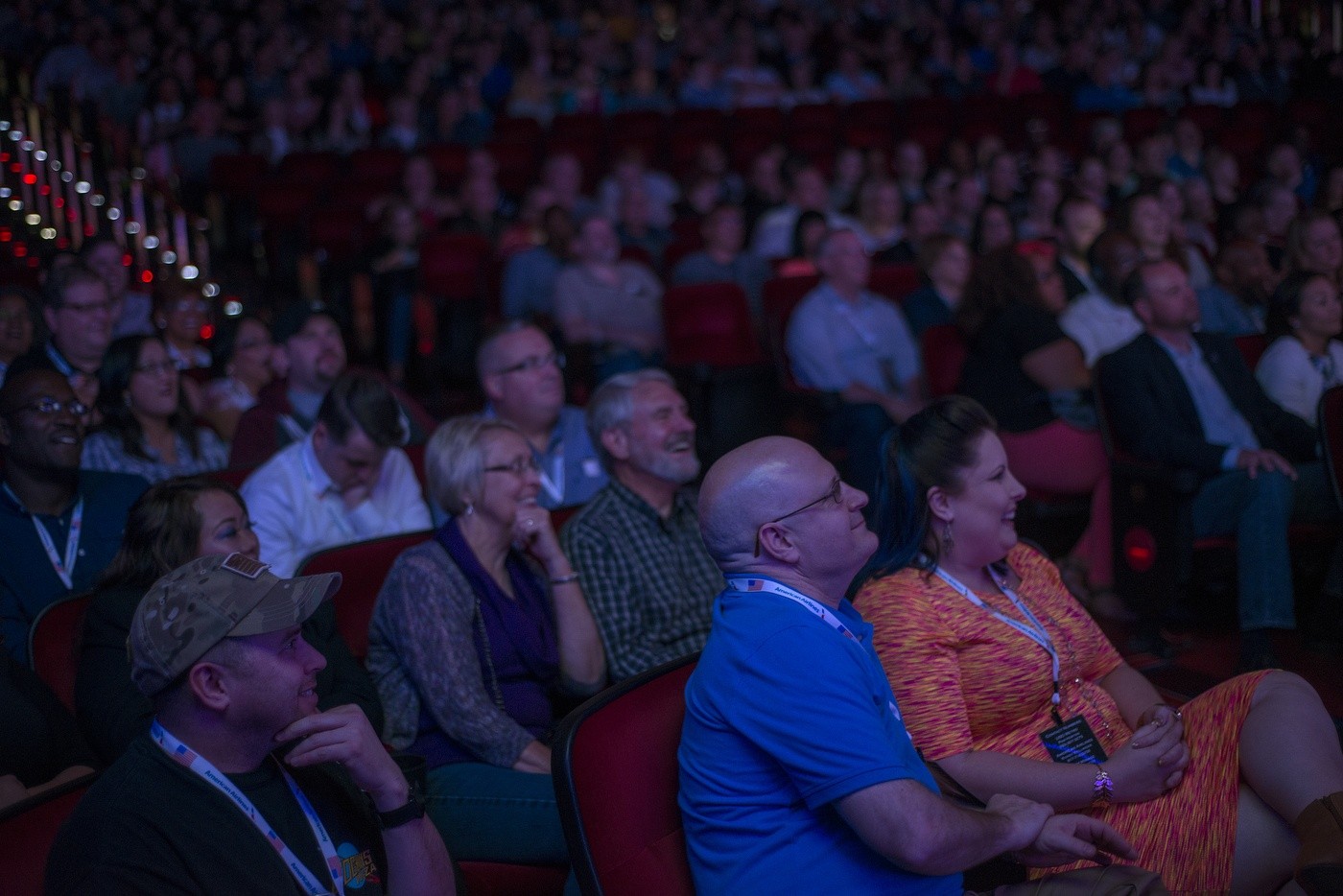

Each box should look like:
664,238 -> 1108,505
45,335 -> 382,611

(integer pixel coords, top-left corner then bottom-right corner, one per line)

175,300 -> 210,312
485,457 -> 539,474
12,396 -> 91,417
132,358 -> 179,375
753,479 -> 844,557
496,347 -> 566,373
66,300 -> 117,313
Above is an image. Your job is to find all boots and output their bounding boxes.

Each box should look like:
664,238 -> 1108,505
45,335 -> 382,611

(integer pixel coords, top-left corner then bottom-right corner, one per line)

1294,791 -> 1343,896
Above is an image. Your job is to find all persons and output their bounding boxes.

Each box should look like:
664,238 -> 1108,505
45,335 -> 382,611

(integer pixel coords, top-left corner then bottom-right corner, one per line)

1106,259 -> 1340,672
0,358 -> 153,789
0,1 -> 1343,579
42,551 -> 457,895
78,476 -> 385,776
851,395 -> 1342,895
679,433 -> 1174,896
555,369 -> 726,680
368,414 -> 610,896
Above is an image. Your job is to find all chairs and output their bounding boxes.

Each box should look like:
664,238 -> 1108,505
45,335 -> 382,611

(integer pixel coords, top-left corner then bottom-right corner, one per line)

264,93 -> 1343,562
3,503 -> 705,895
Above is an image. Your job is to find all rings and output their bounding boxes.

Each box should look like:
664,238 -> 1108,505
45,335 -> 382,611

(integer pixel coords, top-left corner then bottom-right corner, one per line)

527,518 -> 534,527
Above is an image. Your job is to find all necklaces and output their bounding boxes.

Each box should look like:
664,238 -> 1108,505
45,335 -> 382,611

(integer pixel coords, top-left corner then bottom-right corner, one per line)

960,568 -> 1114,741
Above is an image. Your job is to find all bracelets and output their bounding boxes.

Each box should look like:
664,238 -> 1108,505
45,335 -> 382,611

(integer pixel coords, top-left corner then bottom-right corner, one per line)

1153,702 -> 1183,720
549,572 -> 580,584
1092,762 -> 1113,805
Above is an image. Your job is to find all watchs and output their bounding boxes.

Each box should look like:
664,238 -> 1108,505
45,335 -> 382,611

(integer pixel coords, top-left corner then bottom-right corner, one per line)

375,784 -> 424,828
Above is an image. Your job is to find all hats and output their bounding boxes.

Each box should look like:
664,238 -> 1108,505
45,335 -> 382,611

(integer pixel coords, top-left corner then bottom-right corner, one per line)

127,551 -> 343,698
